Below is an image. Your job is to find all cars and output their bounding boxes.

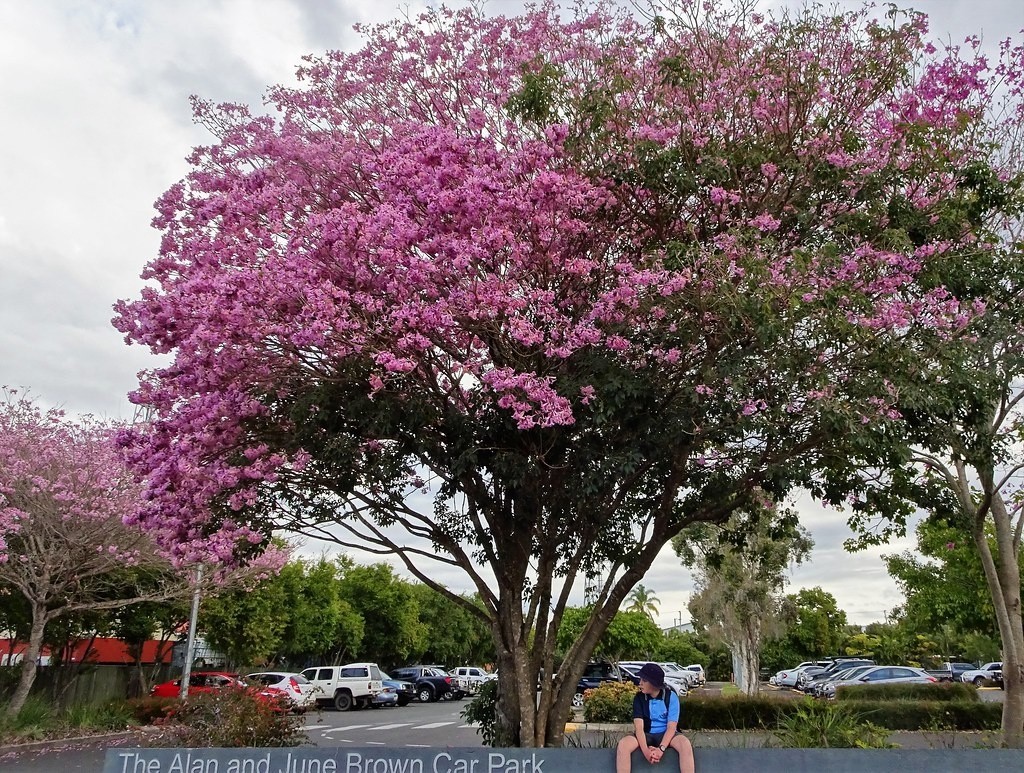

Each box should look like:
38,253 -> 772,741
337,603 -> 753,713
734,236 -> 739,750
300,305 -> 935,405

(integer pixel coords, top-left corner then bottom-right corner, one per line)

770,655 -> 1004,700
238,672 -> 316,715
148,672 -> 293,718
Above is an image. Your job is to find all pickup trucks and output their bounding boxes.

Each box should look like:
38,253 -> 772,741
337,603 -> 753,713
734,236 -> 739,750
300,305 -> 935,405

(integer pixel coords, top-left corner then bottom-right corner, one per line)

389,665 -> 499,703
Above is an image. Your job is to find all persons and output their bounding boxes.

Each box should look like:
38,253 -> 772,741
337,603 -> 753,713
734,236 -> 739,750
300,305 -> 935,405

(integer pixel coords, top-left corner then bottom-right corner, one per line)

617,664 -> 695,772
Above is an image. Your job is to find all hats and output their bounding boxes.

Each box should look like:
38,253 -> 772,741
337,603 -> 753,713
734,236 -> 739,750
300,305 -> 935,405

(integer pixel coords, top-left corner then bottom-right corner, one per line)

635,663 -> 666,688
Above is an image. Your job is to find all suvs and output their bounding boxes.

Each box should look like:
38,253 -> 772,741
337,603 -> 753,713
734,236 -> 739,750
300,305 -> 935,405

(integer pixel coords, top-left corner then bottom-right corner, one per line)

299,663 -> 382,711
369,665 -> 417,709
571,662 -> 705,707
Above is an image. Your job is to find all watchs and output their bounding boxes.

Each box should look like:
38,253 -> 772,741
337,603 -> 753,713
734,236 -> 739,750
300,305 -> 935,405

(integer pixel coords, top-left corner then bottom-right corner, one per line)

659,744 -> 666,752
